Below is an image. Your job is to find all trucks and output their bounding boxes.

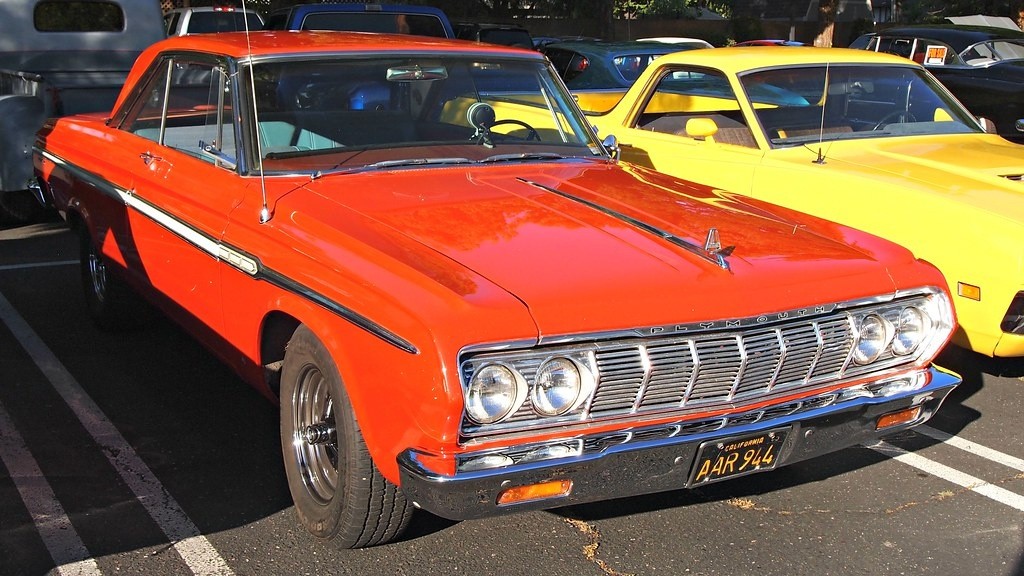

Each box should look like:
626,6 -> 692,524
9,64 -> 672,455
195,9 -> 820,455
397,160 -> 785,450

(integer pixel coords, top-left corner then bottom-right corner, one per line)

261,2 -> 546,122
0,0 -> 195,192
439,46 -> 1024,359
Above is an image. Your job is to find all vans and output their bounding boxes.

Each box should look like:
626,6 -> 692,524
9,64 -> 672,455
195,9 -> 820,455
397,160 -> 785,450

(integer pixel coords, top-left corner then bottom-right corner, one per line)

162,6 -> 264,37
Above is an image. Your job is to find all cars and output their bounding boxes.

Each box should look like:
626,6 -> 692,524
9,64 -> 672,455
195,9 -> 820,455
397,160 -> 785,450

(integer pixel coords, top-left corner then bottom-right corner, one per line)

844,24 -> 1024,133
452,22 -> 536,50
895,58 -> 1024,145
733,40 -> 812,46
536,41 -> 811,107
636,37 -> 716,49
26,30 -> 964,552
